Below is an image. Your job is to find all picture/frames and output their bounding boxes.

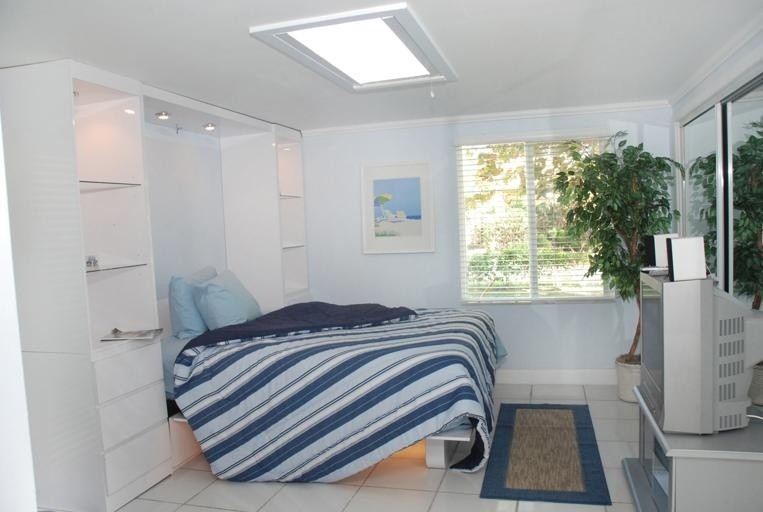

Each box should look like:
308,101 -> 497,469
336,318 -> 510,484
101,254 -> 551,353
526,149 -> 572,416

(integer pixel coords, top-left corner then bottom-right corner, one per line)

361,161 -> 436,254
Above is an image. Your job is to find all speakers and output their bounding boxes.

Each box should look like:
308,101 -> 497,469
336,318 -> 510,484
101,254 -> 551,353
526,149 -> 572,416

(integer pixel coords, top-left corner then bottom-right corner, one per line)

645,232 -> 679,267
667,235 -> 707,282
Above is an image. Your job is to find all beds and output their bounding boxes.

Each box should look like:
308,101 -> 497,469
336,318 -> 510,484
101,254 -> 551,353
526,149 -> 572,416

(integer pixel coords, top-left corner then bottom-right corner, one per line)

162,299 -> 507,482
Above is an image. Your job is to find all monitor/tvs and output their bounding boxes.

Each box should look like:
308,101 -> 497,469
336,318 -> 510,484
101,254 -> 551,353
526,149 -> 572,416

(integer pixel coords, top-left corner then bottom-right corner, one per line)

639,270 -> 763,435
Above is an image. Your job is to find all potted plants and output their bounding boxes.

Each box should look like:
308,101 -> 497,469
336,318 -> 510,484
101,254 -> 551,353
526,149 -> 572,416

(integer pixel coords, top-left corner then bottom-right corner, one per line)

551,130 -> 687,399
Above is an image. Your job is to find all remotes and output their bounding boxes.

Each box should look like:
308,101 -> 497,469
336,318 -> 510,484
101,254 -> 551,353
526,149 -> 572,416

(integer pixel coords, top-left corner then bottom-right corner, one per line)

639,267 -> 668,275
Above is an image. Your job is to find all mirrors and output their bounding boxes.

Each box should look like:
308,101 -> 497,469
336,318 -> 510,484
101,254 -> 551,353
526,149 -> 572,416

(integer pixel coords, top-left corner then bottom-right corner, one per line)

676,61 -> 763,318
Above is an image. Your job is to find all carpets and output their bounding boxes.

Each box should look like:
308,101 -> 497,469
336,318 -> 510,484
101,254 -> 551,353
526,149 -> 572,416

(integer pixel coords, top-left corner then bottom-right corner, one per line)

479,403 -> 613,506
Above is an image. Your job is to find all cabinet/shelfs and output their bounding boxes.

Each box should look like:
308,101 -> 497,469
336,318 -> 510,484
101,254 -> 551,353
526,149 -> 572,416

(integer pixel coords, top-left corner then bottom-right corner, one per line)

221,133 -> 309,310
0,59 -> 175,512
622,386 -> 763,512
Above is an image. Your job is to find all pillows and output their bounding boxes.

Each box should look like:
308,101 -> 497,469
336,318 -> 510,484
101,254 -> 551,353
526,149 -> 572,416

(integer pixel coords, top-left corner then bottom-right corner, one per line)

193,269 -> 263,330
167,264 -> 217,339
